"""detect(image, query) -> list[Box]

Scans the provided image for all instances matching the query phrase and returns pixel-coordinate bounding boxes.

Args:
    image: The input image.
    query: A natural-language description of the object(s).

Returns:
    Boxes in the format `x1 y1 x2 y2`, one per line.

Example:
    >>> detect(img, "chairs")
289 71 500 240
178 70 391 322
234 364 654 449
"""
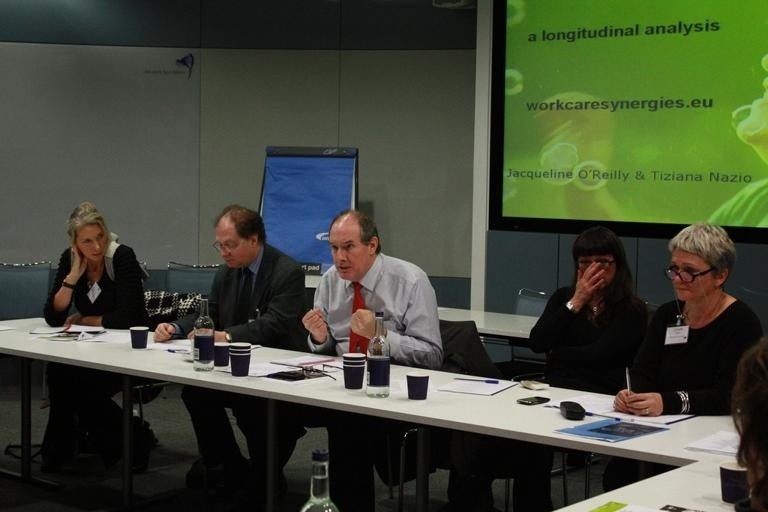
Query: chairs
0 261 768 512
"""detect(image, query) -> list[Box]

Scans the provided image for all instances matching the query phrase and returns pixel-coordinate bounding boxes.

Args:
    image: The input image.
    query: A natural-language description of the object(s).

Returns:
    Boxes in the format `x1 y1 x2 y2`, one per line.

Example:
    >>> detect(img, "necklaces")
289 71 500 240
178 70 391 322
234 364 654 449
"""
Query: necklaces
587 296 604 312
679 288 728 325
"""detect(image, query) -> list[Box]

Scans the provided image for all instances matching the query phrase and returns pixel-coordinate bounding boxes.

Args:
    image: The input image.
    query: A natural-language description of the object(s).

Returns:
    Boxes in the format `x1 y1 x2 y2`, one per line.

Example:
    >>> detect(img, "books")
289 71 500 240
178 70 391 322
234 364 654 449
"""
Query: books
34 318 107 340
273 348 362 372
437 366 520 396
147 329 263 355
545 377 693 444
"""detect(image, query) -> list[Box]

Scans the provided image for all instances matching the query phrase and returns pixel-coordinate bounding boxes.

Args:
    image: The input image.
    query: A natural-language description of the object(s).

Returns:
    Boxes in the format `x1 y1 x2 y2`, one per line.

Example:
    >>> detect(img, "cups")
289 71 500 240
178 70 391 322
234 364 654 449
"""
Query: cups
212 341 229 367
129 326 151 349
719 462 751 504
228 341 254 377
405 371 428 401
341 351 366 390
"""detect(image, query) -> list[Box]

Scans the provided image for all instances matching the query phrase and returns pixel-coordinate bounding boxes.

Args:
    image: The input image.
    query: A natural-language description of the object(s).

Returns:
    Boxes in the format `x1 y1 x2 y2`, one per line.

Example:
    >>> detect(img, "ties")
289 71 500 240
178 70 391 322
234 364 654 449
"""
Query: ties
349 282 369 354
233 267 253 330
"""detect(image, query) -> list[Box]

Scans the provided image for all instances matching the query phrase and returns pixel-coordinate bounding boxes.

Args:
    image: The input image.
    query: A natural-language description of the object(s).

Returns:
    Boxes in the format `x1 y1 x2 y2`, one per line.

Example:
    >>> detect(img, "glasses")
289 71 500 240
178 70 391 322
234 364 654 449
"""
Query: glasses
665 267 714 282
578 260 615 268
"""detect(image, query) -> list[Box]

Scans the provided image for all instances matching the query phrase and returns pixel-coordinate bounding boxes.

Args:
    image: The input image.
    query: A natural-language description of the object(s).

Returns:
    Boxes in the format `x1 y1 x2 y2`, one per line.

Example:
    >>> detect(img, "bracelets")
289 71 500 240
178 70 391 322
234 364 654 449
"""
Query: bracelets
60 281 77 290
677 388 690 414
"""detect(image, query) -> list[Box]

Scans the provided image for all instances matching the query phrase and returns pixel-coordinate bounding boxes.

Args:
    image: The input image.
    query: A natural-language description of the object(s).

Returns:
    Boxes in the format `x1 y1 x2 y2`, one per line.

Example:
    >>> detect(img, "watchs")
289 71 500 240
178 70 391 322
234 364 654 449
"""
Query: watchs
565 300 576 314
224 327 233 343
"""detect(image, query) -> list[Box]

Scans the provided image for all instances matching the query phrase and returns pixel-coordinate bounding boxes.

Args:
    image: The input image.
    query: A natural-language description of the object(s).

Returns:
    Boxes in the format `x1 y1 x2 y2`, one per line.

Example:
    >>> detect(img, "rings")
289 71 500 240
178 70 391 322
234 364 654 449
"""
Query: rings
355 314 361 322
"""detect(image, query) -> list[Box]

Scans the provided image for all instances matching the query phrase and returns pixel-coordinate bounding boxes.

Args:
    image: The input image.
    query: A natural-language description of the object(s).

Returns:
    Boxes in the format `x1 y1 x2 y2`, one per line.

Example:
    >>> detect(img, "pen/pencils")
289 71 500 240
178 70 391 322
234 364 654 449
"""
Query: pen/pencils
453 377 499 384
585 412 620 421
626 367 631 396
168 349 190 354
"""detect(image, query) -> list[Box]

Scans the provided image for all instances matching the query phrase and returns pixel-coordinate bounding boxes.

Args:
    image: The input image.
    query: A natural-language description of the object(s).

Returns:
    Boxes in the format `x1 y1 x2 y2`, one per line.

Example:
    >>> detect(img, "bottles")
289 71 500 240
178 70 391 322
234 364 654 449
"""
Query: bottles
192 295 214 373
299 449 338 512
365 311 391 399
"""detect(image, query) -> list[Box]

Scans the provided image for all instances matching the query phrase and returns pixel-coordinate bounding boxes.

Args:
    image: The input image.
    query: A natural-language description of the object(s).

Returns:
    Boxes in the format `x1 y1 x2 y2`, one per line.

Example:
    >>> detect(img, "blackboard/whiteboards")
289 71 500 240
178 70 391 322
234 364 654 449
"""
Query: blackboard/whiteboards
258 145 359 289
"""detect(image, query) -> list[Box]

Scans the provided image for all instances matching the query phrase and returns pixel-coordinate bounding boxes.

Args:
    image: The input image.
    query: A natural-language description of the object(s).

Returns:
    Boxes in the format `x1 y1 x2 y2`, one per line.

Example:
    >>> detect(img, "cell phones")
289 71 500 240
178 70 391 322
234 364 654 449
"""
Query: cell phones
517 396 550 405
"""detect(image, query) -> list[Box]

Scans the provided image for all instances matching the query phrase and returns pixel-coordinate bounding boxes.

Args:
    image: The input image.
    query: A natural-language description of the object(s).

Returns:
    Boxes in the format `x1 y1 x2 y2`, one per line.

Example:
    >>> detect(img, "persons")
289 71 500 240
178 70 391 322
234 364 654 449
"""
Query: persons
41 200 160 470
730 330 768 510
445 227 651 509
601 220 764 492
151 206 308 500
298 211 443 511
